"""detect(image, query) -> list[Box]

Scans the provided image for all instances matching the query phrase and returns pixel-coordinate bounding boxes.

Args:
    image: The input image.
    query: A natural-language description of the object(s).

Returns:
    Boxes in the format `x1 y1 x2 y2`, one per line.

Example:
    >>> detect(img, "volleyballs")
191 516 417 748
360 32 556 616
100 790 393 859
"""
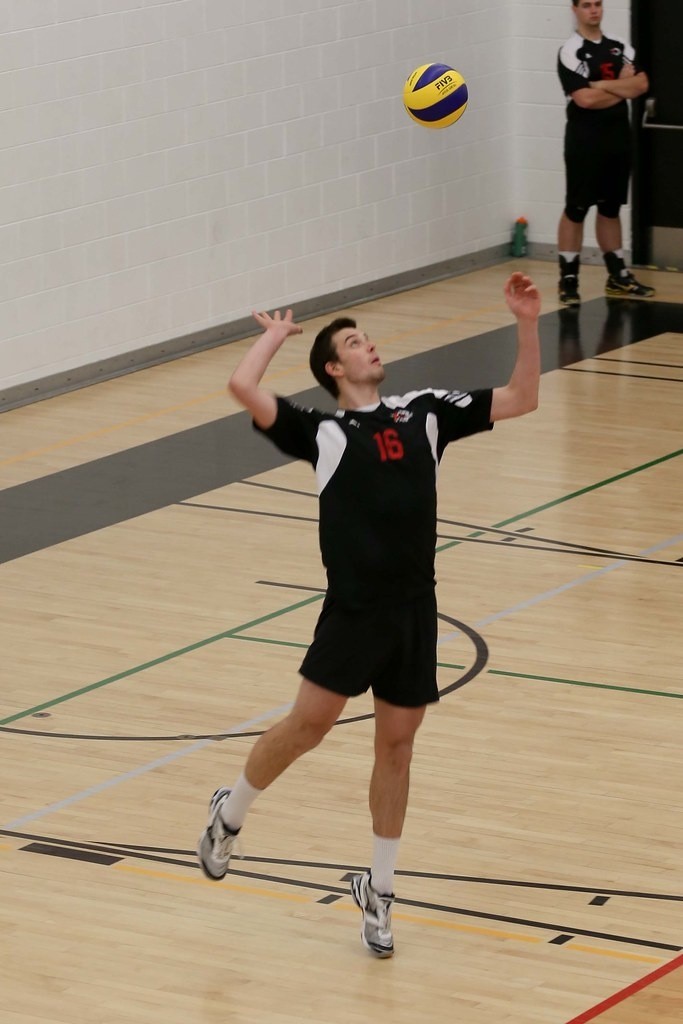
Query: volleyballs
401 61 470 131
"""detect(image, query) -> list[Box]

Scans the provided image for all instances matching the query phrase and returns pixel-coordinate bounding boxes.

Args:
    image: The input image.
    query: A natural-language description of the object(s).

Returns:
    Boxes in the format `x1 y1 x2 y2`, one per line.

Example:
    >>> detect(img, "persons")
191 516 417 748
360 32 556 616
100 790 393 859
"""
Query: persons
197 271 541 958
556 0 664 305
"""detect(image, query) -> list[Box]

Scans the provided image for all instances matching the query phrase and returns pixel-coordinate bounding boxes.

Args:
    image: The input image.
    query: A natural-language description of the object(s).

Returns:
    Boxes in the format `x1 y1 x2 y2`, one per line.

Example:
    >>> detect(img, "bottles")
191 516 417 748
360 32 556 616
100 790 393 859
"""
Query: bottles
512 218 528 256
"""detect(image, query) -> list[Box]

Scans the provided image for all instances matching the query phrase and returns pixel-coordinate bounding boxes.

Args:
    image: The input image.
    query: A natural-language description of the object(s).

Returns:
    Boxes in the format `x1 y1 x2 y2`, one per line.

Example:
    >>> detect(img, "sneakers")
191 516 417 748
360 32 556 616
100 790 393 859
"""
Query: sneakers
349 871 397 959
604 271 655 298
196 787 247 881
557 276 581 306
